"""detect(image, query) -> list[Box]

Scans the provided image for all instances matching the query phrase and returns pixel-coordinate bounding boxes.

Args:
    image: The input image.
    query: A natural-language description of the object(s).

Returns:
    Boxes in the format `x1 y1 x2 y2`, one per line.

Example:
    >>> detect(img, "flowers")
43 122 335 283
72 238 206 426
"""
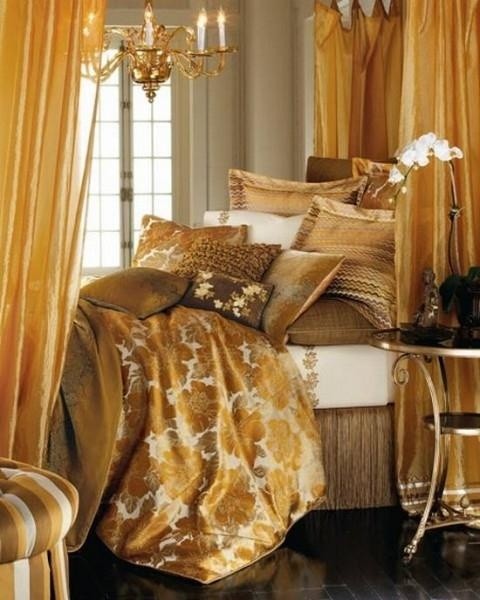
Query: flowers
386 138 464 274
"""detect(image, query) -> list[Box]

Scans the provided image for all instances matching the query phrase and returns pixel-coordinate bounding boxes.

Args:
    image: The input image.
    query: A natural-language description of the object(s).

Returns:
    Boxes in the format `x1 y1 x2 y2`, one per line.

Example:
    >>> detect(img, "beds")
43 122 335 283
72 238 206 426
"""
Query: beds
42 155 415 513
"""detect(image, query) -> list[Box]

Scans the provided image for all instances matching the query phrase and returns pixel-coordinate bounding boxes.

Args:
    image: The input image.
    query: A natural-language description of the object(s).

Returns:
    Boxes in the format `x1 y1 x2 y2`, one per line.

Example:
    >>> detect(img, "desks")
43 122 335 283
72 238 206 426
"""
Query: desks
368 326 480 559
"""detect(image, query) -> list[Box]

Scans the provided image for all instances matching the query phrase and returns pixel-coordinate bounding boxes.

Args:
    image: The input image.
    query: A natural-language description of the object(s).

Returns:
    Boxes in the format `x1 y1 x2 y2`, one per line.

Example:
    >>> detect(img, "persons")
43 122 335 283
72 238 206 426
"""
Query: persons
415 267 441 329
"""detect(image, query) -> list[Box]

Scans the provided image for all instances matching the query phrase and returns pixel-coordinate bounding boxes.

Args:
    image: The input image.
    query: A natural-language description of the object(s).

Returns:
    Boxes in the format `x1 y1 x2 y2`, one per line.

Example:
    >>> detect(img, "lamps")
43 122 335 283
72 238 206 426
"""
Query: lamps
80 0 237 101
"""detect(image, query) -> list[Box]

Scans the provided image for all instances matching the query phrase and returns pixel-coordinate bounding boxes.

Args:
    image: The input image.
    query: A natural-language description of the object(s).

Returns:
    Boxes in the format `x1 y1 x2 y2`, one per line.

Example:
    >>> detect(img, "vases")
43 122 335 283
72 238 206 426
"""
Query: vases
441 265 480 346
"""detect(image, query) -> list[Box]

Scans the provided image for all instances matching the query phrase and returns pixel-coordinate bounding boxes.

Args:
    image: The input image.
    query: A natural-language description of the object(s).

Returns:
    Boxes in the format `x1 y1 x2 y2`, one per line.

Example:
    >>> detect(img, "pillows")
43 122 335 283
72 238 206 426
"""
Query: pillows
170 235 285 280
180 272 278 328
200 157 397 247
248 248 349 344
79 265 189 324
290 195 399 334
130 214 248 278
